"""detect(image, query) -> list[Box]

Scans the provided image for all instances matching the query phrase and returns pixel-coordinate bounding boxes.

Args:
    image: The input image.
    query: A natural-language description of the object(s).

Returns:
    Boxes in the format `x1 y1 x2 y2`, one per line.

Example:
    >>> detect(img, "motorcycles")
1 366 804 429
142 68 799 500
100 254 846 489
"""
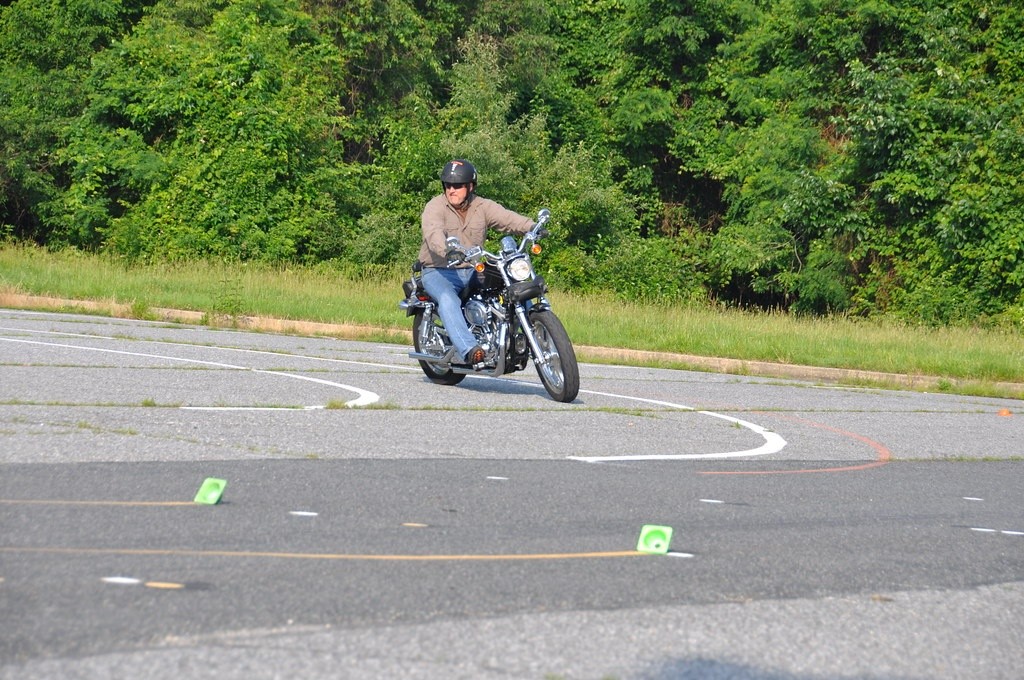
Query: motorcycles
401 207 580 404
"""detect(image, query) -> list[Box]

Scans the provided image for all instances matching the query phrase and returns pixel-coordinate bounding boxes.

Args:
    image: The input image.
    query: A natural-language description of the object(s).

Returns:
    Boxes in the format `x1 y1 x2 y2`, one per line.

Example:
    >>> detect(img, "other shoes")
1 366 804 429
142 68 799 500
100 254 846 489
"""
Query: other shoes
467 346 486 365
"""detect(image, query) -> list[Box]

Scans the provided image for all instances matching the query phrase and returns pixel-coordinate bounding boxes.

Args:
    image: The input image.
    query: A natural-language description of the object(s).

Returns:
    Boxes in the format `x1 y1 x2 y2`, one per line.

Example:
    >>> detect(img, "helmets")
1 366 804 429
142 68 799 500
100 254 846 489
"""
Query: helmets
441 159 477 190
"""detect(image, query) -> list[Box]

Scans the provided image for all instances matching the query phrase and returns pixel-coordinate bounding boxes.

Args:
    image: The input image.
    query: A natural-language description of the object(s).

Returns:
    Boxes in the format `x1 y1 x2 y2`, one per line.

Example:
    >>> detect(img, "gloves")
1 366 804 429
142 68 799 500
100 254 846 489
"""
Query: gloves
447 251 467 265
530 224 549 237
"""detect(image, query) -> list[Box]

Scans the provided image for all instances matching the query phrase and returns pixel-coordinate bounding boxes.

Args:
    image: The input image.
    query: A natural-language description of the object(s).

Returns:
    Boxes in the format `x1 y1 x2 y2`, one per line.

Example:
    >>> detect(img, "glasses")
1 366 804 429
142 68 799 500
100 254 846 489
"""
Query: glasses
443 182 466 189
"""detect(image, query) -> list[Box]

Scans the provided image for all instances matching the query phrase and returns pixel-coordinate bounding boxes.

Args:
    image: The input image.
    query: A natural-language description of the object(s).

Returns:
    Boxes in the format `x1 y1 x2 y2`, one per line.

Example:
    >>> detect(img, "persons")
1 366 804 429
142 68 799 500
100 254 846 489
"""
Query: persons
418 159 550 367
